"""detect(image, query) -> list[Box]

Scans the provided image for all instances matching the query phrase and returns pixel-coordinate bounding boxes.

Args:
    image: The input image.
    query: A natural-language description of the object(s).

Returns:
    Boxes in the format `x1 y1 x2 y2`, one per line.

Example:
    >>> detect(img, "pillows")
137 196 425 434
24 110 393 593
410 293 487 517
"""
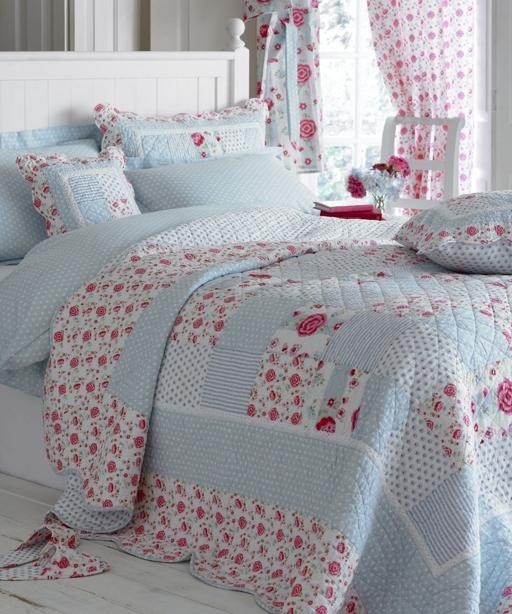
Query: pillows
389 189 511 273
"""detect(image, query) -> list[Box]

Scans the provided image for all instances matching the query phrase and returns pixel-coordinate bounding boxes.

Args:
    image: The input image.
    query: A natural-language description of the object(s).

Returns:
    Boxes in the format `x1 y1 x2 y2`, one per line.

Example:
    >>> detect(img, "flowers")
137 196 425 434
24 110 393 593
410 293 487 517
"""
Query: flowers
342 153 411 212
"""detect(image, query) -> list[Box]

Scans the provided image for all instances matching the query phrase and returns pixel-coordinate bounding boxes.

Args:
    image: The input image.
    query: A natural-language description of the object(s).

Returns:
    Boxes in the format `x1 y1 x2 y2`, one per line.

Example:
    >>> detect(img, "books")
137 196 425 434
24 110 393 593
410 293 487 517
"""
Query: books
311 199 374 213
320 208 382 220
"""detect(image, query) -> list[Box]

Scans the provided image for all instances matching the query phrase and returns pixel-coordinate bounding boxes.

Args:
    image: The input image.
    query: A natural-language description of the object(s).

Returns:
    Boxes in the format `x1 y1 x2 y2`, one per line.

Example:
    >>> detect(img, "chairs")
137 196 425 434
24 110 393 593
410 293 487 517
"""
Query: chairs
380 116 460 214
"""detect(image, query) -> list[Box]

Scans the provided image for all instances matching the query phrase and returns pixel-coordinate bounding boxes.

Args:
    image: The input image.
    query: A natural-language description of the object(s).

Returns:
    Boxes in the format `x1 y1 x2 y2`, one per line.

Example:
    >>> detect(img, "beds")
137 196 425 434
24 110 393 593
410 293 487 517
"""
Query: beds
1 16 512 612
